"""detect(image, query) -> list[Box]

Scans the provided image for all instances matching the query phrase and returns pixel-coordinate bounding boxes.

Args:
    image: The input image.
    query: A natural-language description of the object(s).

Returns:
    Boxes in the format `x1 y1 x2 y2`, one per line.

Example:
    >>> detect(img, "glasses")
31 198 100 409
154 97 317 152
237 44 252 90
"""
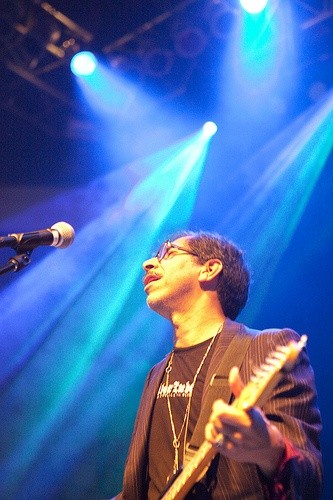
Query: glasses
155 240 208 261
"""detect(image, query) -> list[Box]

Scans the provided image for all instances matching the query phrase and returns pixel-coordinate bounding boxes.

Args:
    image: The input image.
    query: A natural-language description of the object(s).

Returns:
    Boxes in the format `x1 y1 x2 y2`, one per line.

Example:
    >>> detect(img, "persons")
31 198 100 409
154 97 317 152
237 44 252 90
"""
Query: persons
108 229 326 500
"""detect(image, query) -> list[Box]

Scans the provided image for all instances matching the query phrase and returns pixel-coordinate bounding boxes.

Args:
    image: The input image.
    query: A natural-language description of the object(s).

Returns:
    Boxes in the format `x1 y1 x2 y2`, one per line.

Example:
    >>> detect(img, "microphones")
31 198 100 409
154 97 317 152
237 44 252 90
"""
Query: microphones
0 222 75 249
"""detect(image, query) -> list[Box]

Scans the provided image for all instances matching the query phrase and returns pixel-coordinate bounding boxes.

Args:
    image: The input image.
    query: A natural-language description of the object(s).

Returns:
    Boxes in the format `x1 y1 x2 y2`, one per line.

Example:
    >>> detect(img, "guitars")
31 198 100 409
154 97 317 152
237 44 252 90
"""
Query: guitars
159 333 307 500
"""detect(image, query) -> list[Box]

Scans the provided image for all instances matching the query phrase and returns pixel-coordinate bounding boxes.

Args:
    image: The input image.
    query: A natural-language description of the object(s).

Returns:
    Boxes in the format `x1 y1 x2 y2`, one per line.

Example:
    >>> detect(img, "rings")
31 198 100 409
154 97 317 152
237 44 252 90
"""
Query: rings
215 434 224 447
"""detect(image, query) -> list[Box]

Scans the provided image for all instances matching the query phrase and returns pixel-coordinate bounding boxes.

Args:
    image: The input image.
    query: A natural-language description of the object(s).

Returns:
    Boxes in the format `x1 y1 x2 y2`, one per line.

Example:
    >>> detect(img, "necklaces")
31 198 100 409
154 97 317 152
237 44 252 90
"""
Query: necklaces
165 321 223 475
